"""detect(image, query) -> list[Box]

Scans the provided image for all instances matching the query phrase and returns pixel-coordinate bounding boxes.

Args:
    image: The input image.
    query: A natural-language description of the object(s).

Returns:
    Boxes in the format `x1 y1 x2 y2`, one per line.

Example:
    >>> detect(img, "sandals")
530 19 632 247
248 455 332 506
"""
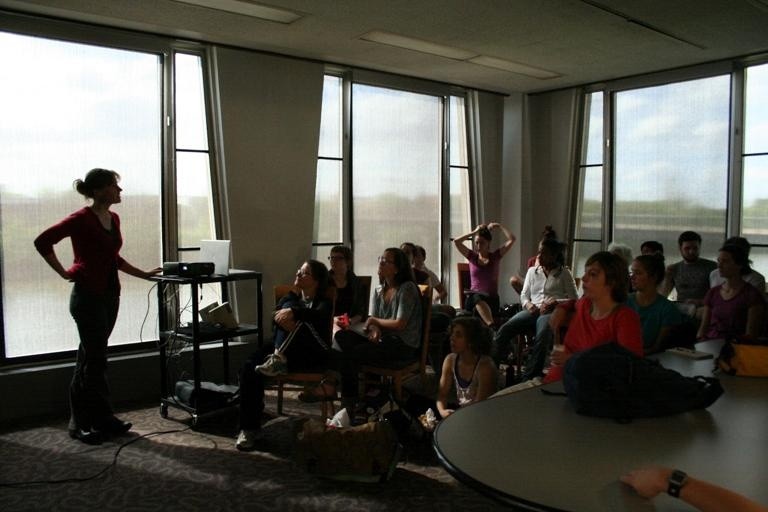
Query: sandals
297 375 337 404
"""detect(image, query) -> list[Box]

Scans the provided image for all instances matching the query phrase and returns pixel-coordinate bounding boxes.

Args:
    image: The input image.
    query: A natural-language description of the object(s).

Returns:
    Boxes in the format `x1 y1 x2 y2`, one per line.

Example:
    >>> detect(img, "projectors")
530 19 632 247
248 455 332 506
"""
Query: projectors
163 261 215 277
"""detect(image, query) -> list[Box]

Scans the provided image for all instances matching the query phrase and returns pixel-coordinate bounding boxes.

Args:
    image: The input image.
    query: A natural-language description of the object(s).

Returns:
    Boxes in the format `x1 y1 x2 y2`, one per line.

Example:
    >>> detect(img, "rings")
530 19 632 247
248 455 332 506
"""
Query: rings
283 315 289 320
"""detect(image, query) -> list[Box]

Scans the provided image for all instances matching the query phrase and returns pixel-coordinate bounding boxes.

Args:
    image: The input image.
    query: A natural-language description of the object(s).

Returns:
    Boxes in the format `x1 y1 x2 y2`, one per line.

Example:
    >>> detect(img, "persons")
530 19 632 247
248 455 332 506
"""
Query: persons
33 169 163 442
434 316 496 419
540 251 643 385
327 245 367 326
232 259 333 449
496 239 579 381
621 465 768 512
297 247 424 423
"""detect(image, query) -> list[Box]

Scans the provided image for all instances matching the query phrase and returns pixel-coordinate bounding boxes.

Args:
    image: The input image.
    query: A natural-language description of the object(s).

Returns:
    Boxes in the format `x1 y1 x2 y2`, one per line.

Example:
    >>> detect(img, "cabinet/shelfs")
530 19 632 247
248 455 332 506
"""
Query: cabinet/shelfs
149 267 267 436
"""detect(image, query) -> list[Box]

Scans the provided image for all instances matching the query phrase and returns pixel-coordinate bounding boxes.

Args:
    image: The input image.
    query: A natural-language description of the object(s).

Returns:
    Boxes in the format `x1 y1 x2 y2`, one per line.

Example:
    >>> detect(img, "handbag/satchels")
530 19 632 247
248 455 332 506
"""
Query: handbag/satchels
717 332 768 375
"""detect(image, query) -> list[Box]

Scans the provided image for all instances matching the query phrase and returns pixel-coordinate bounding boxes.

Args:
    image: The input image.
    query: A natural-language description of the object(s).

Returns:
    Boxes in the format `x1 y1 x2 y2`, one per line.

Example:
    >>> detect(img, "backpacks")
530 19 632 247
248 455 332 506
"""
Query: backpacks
564 343 724 422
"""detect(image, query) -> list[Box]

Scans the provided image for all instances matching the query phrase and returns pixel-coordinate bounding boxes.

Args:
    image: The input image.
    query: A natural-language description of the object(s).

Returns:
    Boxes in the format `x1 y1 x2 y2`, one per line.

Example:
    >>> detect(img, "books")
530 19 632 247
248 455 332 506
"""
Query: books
208 301 237 329
198 299 219 322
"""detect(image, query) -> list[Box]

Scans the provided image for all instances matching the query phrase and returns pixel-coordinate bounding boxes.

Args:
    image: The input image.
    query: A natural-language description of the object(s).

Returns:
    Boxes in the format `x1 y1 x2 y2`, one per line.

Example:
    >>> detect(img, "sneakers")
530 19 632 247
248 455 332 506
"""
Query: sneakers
238 429 267 452
254 356 289 378
68 421 133 444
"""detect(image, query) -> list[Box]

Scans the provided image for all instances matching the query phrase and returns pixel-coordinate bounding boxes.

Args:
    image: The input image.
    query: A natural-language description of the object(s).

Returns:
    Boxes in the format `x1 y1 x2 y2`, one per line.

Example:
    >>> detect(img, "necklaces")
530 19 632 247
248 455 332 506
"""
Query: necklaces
92 208 110 216
595 306 609 320
334 274 346 284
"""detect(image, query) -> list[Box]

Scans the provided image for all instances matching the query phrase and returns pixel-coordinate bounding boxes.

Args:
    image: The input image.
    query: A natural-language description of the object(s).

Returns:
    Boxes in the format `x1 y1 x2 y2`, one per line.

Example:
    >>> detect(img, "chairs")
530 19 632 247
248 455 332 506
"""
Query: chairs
666 300 710 345
256 262 535 430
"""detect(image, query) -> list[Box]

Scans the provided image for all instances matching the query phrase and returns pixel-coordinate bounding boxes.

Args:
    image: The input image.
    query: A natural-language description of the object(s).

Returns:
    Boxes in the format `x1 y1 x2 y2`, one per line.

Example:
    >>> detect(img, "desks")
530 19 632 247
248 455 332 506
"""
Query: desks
431 338 767 512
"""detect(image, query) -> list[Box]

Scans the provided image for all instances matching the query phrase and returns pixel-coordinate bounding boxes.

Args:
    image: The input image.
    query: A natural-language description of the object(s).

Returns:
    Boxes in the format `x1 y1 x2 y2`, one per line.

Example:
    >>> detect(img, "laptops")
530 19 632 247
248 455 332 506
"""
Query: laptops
199 240 255 276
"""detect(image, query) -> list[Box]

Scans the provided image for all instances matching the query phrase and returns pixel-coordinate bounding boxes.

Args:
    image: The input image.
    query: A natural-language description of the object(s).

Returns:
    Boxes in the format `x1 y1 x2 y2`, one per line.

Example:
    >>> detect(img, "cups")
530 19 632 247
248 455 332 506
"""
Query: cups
550 344 566 367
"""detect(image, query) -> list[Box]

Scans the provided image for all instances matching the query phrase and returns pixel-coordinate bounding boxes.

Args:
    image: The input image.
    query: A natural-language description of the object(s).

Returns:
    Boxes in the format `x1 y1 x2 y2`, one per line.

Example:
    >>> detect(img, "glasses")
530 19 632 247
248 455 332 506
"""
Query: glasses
296 269 313 276
327 255 345 261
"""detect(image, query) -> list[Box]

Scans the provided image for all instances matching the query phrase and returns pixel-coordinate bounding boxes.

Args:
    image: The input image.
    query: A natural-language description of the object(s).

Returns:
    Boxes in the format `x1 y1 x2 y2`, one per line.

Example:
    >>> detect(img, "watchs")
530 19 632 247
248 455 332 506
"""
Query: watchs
667 469 688 497
